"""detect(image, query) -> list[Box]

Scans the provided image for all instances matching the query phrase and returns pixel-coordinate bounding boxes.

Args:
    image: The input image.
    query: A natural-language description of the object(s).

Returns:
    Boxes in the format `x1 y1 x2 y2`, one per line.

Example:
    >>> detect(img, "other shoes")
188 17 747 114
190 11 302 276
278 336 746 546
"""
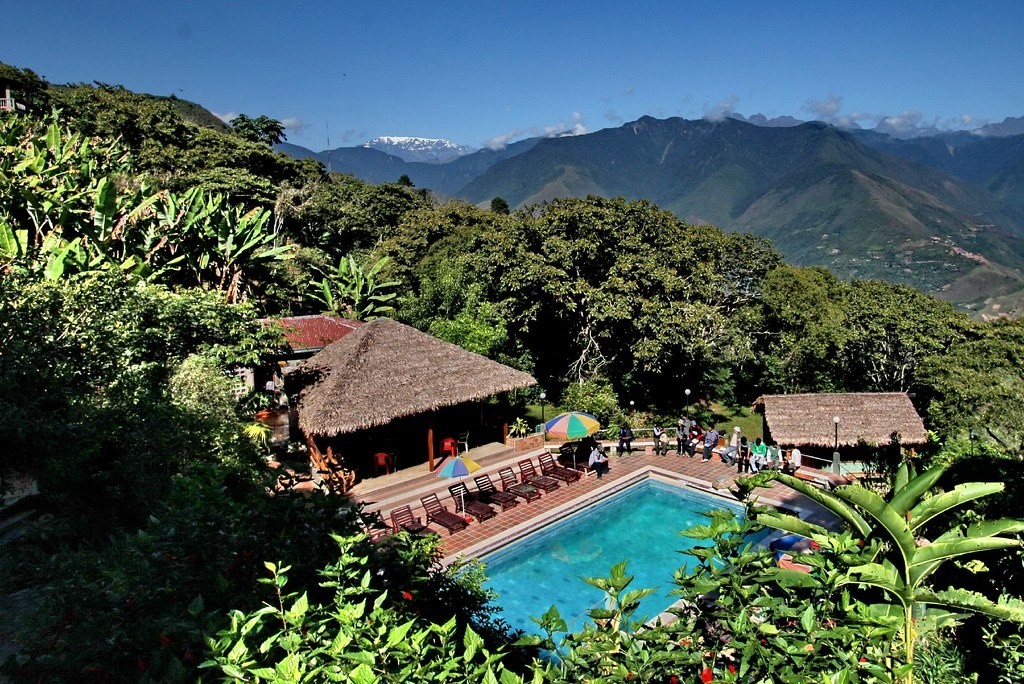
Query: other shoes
597 475 603 479
656 452 660 456
701 458 708 463
627 450 633 456
750 471 757 474
605 471 611 474
682 454 685 457
676 454 679 457
662 453 666 457
619 451 623 458
725 461 732 467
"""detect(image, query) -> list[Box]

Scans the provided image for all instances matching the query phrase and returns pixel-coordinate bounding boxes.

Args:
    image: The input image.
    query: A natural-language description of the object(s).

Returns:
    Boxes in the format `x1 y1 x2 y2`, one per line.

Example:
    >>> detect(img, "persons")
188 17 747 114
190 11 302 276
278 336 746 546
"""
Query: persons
653 419 802 474
588 444 607 479
618 420 632 456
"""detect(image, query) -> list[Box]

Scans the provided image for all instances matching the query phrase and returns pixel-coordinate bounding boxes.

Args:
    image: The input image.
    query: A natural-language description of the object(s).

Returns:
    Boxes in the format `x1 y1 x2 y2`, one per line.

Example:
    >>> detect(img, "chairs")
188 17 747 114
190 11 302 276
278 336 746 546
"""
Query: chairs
538 451 580 486
448 482 495 523
559 445 596 476
474 474 517 512
365 514 396 545
373 452 392 475
518 458 559 494
314 455 354 490
391 504 437 536
457 431 469 453
498 467 540 504
438 437 456 457
420 493 466 535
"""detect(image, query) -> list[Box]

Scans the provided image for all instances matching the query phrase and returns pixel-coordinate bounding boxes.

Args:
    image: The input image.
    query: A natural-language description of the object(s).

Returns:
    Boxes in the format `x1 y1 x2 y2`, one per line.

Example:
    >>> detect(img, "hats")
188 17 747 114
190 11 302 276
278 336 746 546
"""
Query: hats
690 420 697 426
677 419 684 425
733 425 741 433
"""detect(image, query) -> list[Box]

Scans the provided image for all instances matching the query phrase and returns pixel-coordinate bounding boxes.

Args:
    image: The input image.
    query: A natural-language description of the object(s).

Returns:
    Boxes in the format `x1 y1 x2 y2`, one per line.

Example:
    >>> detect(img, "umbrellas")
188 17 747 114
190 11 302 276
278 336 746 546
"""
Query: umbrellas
436 457 481 522
545 410 601 473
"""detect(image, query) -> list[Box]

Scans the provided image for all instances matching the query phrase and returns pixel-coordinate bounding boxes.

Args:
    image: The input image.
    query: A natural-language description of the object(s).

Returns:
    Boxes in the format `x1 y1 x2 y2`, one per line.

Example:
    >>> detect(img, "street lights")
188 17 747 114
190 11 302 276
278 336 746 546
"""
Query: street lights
539 392 547 423
628 400 635 430
832 416 840 452
683 389 691 419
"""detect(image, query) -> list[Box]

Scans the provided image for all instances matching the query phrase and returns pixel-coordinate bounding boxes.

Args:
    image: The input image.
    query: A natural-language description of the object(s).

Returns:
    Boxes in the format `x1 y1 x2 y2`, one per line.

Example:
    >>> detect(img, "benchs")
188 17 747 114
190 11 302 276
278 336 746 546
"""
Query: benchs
543 438 849 489
